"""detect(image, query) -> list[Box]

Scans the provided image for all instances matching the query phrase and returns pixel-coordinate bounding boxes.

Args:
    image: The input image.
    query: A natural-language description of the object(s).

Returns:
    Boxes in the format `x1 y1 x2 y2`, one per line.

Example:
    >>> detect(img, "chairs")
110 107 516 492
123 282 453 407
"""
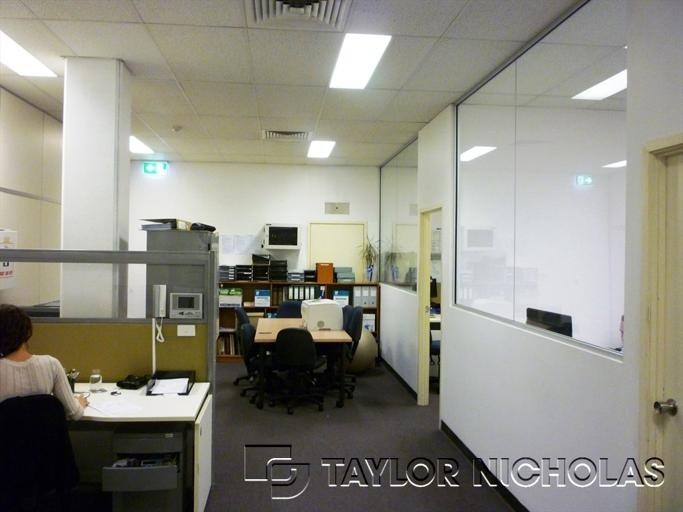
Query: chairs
0 393 79 511
232 299 363 415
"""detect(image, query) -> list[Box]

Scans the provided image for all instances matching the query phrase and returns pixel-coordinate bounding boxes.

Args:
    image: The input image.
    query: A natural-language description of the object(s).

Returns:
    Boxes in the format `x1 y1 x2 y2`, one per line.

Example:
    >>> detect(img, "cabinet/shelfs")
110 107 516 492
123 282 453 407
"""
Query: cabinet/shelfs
100 429 184 511
216 281 380 364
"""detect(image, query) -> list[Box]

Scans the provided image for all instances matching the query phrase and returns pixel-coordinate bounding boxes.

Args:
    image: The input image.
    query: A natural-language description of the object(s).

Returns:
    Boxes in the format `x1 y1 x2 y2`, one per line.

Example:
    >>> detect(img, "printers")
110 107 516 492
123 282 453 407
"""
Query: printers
301 298 343 330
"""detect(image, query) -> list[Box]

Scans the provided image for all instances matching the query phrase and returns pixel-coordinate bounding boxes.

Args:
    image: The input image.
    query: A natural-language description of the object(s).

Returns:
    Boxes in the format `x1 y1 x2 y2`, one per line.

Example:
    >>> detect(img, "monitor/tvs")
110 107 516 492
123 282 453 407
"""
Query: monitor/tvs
264 224 302 250
527 307 572 336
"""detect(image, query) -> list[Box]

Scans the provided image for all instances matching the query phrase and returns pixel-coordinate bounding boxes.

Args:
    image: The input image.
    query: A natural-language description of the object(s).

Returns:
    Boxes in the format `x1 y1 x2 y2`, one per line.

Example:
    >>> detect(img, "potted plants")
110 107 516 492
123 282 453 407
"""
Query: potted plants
354 232 379 282
383 237 400 283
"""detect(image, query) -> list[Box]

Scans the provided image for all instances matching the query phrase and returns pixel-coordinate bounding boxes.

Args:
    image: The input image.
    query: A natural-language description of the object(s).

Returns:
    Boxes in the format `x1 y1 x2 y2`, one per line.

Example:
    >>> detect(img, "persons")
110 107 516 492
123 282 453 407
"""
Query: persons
0 304 90 423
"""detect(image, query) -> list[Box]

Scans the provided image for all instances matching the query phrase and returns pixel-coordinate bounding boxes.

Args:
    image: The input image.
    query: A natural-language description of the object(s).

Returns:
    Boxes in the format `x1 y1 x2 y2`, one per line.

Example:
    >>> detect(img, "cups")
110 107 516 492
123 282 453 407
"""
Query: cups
67 377 75 394
88 374 103 391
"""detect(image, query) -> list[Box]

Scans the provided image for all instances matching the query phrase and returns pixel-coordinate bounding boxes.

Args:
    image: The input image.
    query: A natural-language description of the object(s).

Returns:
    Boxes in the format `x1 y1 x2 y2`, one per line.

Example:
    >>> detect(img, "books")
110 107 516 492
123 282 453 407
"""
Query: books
217 333 236 356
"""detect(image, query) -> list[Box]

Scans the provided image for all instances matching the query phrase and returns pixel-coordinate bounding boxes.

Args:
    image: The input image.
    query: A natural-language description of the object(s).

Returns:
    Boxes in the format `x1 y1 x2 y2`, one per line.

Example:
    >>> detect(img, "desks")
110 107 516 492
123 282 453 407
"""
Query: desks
51 379 212 511
